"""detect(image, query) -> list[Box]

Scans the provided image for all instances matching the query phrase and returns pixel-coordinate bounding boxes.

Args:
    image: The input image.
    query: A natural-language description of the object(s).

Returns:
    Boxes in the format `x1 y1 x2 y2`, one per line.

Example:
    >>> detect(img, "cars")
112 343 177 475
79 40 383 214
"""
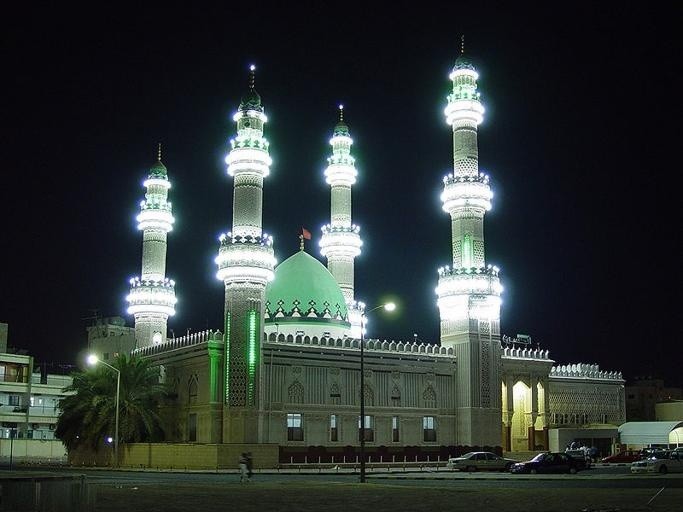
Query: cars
507 450 577 476
560 445 683 476
447 450 521 473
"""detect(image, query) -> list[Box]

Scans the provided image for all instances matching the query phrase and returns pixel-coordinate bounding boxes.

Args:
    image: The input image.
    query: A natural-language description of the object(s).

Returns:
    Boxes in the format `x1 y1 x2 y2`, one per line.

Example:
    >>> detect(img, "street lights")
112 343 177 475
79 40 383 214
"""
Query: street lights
5 422 14 471
356 302 398 484
86 353 122 470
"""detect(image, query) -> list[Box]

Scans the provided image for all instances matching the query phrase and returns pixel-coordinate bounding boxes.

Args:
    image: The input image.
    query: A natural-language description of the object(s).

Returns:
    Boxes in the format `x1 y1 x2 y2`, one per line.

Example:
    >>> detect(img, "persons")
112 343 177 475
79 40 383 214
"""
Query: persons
238 451 248 483
564 440 597 465
246 451 253 482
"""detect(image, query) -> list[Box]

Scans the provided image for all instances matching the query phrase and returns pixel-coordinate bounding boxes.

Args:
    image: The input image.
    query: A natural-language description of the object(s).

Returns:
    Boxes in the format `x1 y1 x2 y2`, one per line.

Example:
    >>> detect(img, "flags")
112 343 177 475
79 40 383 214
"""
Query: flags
303 228 312 240
